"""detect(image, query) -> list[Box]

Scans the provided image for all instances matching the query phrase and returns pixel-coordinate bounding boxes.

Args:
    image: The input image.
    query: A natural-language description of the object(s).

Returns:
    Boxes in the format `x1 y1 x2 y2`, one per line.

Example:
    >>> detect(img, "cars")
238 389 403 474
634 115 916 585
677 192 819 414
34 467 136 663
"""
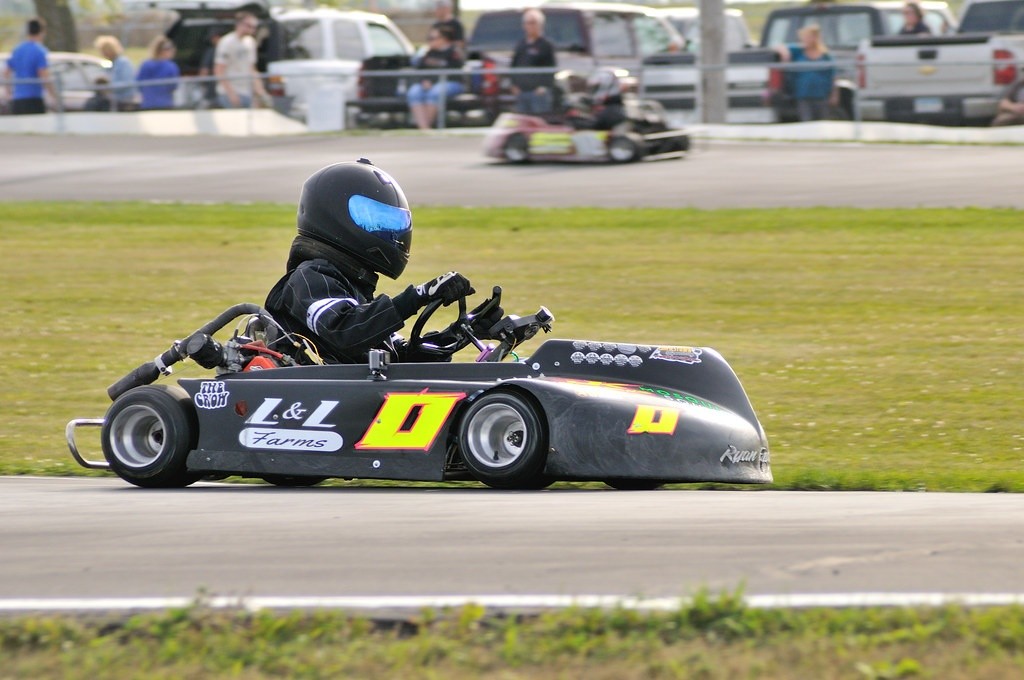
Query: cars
0 52 142 110
658 9 750 54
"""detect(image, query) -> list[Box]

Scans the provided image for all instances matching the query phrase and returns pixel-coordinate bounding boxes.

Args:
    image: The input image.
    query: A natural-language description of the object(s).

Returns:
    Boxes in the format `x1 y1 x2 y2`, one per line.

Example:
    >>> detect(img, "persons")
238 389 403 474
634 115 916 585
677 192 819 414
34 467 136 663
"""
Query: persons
537 67 634 129
900 3 930 34
265 157 505 365
994 66 1024 126
84 77 111 112
137 39 179 110
198 29 226 77
769 23 838 122
98 36 135 112
5 20 60 115
214 12 272 109
508 9 555 114
407 0 466 128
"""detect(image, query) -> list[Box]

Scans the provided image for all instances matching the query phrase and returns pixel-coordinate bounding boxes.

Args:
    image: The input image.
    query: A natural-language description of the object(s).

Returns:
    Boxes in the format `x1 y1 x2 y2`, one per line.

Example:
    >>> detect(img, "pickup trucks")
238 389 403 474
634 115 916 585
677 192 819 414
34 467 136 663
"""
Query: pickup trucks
854 0 1024 121
639 0 957 107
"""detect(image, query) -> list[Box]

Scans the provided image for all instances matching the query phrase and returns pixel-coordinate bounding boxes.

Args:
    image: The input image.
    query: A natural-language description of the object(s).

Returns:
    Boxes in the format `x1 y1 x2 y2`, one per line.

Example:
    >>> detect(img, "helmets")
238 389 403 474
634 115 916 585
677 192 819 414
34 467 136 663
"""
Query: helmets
584 65 628 102
297 158 412 281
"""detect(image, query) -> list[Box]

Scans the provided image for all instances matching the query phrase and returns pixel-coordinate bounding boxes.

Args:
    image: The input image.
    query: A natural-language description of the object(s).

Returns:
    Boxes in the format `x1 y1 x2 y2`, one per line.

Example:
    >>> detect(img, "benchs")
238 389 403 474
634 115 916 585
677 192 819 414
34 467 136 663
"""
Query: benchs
342 50 560 129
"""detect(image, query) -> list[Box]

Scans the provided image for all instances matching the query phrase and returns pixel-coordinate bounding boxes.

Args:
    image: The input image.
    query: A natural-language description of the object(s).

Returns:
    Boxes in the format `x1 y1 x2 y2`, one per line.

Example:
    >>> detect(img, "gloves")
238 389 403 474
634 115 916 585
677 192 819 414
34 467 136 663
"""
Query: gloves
460 298 503 340
416 272 470 306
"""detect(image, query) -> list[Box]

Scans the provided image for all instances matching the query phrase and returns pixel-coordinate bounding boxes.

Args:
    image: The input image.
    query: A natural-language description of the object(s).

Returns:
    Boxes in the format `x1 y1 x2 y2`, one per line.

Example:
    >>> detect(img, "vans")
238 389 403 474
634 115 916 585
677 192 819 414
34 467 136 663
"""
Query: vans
145 0 415 132
471 4 690 101
